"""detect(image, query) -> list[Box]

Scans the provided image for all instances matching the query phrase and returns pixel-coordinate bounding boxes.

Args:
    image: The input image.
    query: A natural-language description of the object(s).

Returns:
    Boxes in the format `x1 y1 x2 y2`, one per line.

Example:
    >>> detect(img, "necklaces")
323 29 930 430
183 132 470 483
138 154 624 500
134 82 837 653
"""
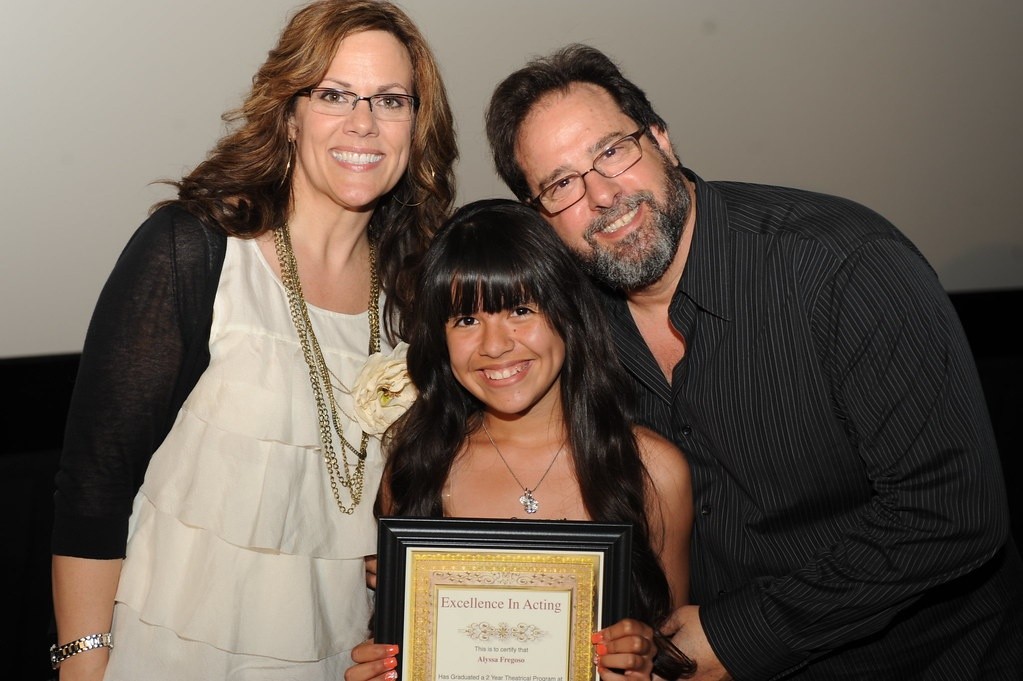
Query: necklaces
478 422 567 514
270 216 382 516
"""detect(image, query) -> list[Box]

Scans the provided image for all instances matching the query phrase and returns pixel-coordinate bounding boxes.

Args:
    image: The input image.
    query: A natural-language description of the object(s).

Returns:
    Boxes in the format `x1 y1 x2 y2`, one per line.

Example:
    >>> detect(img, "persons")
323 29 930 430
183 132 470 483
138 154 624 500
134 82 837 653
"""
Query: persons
343 200 692 681
487 43 1023 681
51 0 460 681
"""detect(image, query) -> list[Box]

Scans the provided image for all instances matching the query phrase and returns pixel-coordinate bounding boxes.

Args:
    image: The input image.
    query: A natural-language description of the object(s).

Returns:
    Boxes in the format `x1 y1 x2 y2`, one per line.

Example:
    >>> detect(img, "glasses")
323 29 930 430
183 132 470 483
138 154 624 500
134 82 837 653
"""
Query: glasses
530 124 648 217
300 86 420 122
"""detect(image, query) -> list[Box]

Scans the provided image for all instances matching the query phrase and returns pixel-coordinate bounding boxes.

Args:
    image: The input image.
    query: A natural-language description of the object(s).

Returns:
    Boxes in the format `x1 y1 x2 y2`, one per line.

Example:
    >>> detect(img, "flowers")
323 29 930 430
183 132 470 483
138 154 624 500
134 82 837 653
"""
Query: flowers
350 341 420 445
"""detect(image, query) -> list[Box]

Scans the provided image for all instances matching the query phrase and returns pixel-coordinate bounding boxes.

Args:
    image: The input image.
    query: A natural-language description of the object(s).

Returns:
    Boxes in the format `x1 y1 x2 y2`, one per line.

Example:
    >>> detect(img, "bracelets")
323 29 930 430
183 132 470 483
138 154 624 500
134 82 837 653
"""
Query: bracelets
50 633 114 670
